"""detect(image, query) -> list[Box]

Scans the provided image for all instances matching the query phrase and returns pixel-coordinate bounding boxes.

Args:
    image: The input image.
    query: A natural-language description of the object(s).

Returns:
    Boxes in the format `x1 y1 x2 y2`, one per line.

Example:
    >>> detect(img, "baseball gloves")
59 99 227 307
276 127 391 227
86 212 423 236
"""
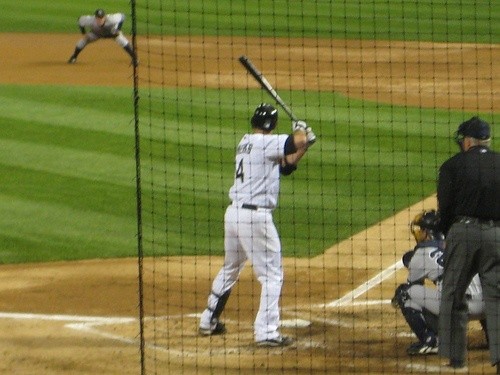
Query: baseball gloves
391 283 411 309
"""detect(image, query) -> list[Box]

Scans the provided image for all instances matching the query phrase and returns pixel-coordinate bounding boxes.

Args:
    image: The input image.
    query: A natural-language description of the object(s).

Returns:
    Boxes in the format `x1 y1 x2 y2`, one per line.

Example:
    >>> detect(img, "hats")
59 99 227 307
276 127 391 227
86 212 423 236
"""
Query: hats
95 9 105 17
457 116 492 140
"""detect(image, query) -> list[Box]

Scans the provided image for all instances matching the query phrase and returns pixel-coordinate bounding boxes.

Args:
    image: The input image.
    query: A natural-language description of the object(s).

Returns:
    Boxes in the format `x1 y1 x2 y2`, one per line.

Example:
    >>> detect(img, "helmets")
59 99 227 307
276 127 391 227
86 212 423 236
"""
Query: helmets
410 207 447 244
250 102 279 130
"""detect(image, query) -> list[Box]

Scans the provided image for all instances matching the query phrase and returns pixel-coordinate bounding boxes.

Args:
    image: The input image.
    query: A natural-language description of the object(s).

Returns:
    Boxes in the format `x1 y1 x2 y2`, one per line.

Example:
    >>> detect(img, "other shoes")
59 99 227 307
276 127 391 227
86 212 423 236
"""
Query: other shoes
403 341 441 357
67 55 76 64
198 321 225 334
256 332 293 347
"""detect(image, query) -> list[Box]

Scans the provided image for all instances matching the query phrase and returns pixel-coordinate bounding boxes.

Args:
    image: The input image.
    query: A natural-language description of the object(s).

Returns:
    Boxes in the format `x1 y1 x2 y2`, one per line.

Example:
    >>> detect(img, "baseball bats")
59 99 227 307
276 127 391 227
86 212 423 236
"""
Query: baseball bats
237 54 316 144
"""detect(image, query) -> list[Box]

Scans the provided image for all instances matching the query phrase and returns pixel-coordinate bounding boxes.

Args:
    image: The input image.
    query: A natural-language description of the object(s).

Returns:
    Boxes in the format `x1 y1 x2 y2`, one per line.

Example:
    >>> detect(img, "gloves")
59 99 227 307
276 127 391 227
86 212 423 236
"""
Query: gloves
304 126 317 145
292 119 307 130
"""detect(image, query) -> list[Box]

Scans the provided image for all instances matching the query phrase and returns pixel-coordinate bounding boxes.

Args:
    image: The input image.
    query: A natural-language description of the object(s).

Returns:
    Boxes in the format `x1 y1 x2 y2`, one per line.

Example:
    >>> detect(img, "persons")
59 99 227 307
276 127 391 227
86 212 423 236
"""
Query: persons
67 9 140 67
197 101 316 348
392 209 491 357
434 116 500 375
